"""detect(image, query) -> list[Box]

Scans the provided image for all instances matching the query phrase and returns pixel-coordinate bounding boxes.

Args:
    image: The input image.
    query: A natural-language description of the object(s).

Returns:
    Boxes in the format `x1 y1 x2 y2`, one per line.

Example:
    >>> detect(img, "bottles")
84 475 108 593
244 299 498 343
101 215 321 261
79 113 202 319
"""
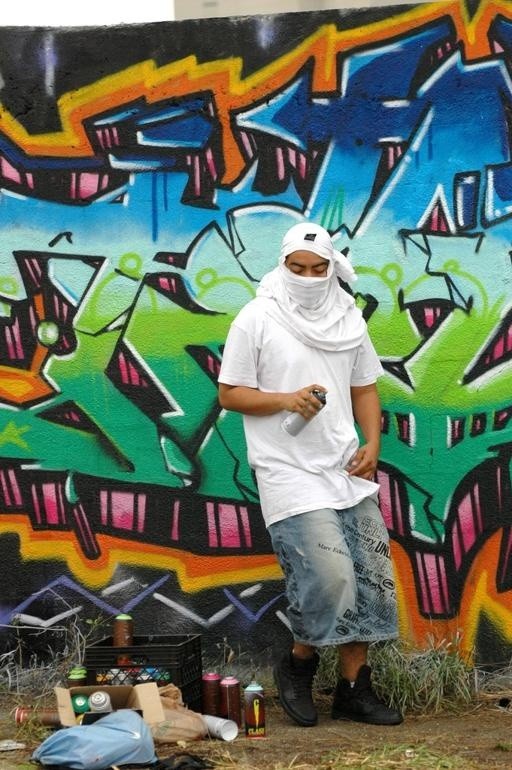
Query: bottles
112 612 134 651
198 668 266 743
15 708 61 726
281 389 328 437
70 659 170 713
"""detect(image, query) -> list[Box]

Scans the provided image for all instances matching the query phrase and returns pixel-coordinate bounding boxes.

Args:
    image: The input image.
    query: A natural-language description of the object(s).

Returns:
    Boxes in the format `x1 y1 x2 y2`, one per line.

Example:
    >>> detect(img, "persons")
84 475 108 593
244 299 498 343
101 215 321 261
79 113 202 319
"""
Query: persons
214 219 408 728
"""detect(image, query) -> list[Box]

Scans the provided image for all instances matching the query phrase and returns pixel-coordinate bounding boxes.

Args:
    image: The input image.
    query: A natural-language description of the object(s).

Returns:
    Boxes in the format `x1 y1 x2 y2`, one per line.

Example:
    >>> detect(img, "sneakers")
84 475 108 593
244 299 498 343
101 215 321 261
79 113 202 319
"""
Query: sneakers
329 662 405 727
271 643 322 728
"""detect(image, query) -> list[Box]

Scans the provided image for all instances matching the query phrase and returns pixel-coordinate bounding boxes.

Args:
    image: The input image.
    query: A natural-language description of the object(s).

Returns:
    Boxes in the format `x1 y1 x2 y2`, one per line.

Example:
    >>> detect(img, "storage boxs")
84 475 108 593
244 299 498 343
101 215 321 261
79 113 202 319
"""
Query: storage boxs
54 679 165 729
